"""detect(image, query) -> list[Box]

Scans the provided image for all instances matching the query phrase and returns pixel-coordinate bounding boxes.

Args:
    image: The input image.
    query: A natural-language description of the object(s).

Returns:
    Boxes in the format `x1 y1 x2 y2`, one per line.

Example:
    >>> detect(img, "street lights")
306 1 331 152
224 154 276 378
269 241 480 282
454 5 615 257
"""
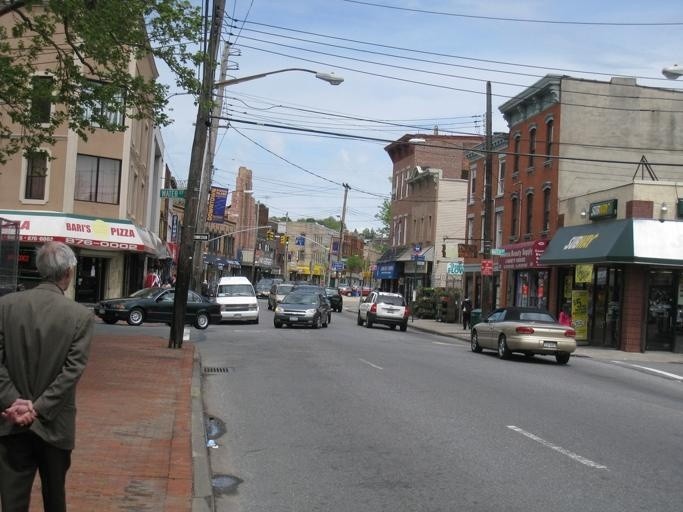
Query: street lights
662 63 683 80
192 68 345 294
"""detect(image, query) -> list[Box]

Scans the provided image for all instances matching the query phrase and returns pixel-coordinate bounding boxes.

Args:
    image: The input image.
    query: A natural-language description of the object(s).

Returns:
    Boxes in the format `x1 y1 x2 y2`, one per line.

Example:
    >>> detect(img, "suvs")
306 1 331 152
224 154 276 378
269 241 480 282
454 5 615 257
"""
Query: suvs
358 289 410 331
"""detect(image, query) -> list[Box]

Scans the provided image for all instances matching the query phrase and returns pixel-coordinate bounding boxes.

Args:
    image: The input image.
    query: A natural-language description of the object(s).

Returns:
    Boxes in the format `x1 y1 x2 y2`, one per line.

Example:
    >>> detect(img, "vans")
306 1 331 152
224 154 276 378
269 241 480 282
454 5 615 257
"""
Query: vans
215 276 260 324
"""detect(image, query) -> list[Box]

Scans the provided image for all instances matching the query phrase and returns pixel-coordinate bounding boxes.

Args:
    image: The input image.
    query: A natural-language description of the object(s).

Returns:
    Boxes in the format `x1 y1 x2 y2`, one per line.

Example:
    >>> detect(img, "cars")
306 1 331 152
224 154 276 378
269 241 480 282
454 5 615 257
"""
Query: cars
339 284 374 296
256 284 342 329
94 287 222 329
470 307 578 365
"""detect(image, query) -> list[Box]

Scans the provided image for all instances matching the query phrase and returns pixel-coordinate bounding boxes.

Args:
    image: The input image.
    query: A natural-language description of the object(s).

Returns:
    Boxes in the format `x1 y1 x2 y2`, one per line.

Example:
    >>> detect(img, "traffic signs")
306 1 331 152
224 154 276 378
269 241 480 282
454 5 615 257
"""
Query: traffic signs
160 189 186 199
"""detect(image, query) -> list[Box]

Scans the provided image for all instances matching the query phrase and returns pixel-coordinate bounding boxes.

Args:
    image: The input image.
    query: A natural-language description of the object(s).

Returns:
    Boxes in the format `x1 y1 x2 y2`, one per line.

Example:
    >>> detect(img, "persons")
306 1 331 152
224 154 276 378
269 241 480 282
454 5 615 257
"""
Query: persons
558 304 570 326
461 296 471 330
0 241 94 511
